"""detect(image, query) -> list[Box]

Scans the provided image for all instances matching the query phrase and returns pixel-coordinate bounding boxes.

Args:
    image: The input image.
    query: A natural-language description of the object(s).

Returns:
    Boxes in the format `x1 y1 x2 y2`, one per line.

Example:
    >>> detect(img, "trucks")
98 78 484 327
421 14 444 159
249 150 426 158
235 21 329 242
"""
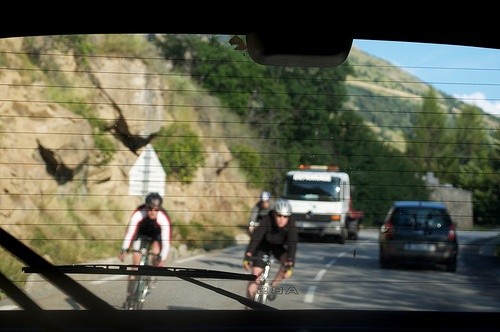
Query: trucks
277 164 365 244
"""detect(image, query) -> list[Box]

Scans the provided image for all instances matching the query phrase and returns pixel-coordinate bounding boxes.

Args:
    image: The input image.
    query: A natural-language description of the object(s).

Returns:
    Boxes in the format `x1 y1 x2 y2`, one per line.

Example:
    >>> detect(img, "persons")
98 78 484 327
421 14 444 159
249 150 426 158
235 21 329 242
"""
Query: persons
121 192 171 309
243 199 297 310
247 191 273 237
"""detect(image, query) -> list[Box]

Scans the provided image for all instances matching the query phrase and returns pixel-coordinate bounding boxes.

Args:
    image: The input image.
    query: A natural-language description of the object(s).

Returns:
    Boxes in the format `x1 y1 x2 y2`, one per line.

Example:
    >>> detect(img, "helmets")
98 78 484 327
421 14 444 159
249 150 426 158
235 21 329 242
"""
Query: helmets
260 191 270 201
146 192 162 208
274 198 292 216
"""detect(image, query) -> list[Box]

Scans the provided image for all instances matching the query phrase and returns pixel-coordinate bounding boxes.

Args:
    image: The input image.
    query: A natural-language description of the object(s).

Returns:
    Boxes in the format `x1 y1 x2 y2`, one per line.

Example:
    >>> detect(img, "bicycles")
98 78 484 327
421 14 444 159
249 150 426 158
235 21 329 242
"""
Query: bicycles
242 254 287 307
119 247 159 310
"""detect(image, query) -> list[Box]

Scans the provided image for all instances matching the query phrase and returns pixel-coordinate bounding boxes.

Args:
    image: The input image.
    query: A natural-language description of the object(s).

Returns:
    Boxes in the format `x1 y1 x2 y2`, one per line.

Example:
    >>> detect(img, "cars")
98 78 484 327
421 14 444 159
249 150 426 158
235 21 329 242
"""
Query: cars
377 201 459 272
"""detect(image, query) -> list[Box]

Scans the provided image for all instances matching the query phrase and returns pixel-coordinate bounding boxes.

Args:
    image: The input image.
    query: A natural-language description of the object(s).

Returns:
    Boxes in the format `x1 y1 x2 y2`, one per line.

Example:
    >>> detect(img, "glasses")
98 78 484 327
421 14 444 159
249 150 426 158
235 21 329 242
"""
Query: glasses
277 214 288 218
147 209 159 211
